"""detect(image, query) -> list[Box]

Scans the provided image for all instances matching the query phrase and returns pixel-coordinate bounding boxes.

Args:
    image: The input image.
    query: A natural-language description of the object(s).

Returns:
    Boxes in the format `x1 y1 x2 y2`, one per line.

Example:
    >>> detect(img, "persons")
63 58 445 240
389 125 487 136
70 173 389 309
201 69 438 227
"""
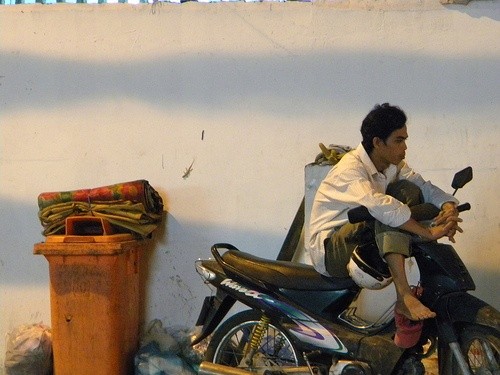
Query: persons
306 102 463 322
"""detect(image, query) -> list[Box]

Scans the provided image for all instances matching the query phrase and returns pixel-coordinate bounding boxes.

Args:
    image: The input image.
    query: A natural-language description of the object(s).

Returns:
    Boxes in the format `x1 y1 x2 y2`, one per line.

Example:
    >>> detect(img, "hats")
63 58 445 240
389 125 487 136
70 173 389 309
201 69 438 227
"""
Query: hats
394 287 426 349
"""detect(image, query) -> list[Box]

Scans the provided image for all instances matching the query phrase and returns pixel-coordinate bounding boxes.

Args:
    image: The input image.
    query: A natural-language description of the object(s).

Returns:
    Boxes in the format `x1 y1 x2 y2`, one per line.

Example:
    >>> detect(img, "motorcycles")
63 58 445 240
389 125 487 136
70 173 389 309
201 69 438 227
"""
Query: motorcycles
188 166 500 375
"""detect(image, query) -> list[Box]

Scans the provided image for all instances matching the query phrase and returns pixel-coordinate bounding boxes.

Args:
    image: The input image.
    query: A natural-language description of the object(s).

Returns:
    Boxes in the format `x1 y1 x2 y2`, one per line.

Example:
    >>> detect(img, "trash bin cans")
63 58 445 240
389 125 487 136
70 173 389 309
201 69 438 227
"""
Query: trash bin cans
30 215 150 375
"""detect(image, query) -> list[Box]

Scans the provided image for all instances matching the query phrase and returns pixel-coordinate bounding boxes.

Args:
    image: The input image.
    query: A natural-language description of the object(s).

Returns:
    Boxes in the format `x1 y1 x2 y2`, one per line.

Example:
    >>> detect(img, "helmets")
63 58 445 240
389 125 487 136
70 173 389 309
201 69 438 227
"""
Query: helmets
348 246 394 290
